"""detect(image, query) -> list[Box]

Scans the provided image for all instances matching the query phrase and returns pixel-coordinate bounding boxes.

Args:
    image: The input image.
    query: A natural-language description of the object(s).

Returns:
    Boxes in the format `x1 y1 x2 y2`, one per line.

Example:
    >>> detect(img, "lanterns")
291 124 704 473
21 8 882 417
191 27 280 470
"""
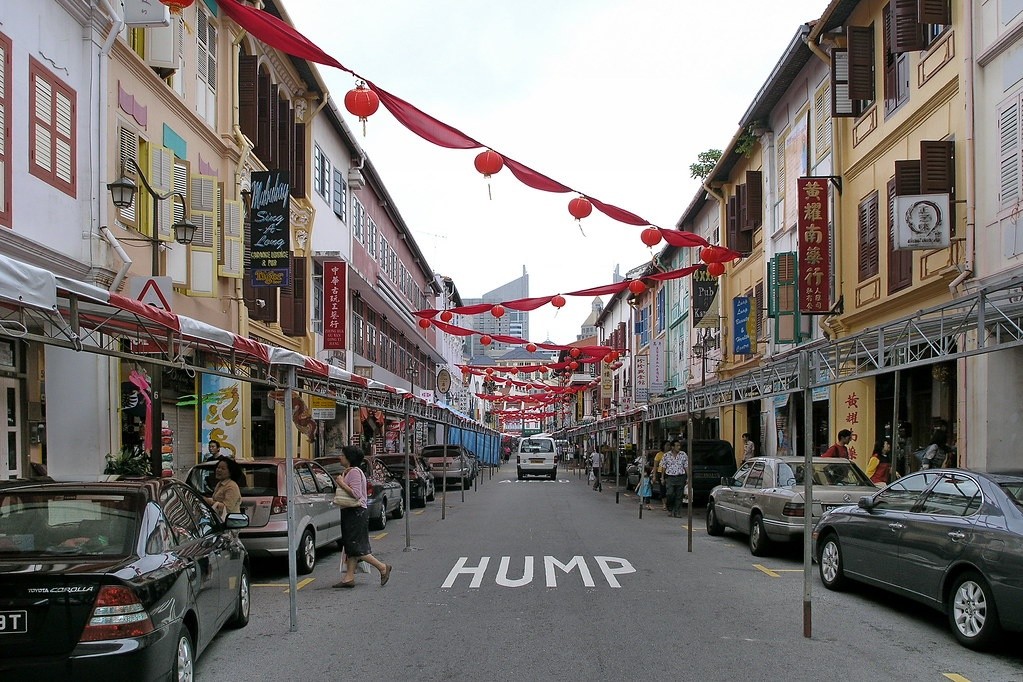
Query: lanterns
568 196 592 238
641 228 662 256
160 0 196 34
419 247 726 423
344 86 379 137
474 150 504 200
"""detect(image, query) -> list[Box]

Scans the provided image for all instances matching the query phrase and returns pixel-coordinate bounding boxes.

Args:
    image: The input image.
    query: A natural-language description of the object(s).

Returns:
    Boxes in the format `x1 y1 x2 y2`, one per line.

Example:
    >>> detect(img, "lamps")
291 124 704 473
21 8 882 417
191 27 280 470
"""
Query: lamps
667 387 677 395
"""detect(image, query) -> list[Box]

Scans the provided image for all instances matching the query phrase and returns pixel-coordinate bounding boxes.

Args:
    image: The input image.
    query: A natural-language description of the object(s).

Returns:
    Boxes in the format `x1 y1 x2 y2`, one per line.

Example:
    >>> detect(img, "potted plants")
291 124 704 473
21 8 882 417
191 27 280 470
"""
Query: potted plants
100 443 153 485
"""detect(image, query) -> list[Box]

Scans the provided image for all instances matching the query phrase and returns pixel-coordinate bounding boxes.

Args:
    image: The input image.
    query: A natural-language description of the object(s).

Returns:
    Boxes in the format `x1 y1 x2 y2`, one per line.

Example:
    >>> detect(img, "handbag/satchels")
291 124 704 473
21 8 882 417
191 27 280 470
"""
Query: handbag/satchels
340 547 371 574
204 564 213 582
334 468 363 508
913 445 938 467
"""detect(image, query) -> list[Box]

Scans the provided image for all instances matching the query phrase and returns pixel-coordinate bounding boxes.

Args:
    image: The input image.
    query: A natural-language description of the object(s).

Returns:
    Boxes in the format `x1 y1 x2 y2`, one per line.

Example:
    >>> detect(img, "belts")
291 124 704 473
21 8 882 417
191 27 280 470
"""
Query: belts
668 475 685 477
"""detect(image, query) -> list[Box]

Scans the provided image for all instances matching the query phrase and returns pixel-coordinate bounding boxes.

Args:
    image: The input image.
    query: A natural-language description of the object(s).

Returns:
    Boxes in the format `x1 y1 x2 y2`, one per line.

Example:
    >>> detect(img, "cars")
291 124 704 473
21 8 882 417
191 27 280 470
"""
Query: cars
625 449 661 495
184 457 343 575
313 457 406 529
705 455 882 558
811 466 1023 651
0 478 252 682
375 454 436 507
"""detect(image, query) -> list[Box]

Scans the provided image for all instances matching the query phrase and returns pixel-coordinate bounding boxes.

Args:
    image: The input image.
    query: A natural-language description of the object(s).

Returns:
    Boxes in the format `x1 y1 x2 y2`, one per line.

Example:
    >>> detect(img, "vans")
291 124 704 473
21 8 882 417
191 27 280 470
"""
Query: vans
516 436 558 480
680 440 737 504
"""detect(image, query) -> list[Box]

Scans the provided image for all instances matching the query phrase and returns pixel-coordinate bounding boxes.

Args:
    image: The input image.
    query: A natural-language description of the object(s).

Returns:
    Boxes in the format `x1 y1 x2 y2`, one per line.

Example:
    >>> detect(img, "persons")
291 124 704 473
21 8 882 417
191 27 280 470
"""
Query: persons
919 430 957 472
822 429 851 484
330 446 393 589
555 433 755 518
200 440 243 540
864 440 902 489
502 441 519 464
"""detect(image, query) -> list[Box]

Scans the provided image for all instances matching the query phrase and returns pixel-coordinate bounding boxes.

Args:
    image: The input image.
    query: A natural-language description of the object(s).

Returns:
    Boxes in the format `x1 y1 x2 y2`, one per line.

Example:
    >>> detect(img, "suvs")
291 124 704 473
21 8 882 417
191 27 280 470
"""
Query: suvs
421 444 475 490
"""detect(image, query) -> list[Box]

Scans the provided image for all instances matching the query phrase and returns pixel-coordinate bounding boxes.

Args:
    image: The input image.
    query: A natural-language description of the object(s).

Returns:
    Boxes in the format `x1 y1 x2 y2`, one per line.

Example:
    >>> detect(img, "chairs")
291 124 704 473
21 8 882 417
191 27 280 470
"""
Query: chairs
253 473 277 494
0 510 131 556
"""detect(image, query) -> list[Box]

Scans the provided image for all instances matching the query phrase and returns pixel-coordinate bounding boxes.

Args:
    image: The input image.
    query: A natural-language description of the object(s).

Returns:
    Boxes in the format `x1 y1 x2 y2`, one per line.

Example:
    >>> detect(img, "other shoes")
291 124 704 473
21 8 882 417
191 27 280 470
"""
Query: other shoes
332 580 356 588
642 504 654 511
662 504 667 511
381 565 392 586
592 488 602 491
668 512 682 518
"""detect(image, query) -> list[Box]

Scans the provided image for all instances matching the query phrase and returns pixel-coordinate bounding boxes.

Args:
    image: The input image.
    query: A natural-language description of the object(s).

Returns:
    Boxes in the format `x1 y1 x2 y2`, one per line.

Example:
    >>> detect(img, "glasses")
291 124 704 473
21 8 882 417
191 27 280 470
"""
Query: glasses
337 454 345 458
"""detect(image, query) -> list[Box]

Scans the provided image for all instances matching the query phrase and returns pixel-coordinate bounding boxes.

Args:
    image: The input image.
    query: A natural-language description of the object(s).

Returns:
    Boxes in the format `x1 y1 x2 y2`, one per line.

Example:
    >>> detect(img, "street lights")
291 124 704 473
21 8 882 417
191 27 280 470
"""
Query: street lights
622 379 633 449
691 325 715 440
107 154 198 476
406 363 419 454
